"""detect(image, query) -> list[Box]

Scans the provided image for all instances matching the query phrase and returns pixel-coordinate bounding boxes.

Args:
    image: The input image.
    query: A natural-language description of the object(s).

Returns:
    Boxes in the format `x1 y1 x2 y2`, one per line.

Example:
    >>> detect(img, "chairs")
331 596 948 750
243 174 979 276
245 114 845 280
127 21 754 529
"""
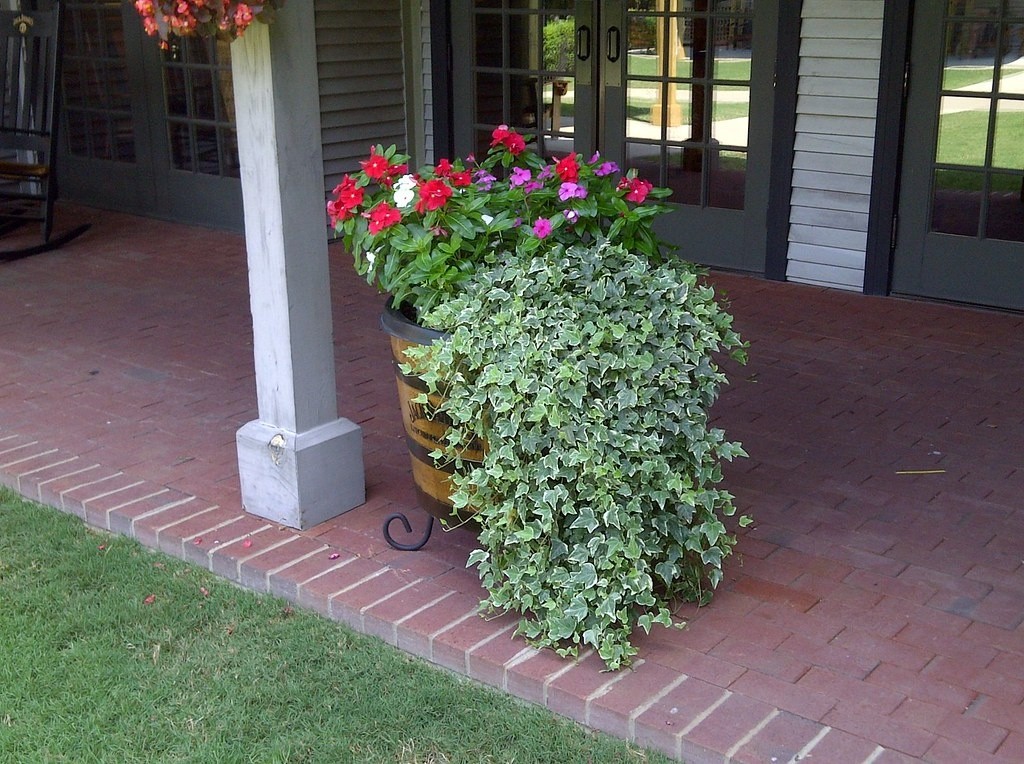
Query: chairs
0 3 92 262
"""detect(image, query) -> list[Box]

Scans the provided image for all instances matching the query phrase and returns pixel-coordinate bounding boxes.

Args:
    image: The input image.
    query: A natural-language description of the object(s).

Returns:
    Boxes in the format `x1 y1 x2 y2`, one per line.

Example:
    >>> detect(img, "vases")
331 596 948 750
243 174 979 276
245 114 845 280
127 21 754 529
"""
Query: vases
378 295 500 531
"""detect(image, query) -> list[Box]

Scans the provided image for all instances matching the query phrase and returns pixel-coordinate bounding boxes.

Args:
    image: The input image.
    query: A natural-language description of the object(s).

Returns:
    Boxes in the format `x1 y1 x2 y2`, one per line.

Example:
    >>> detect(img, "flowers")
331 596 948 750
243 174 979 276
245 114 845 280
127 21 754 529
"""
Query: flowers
134 0 285 50
325 125 676 322
